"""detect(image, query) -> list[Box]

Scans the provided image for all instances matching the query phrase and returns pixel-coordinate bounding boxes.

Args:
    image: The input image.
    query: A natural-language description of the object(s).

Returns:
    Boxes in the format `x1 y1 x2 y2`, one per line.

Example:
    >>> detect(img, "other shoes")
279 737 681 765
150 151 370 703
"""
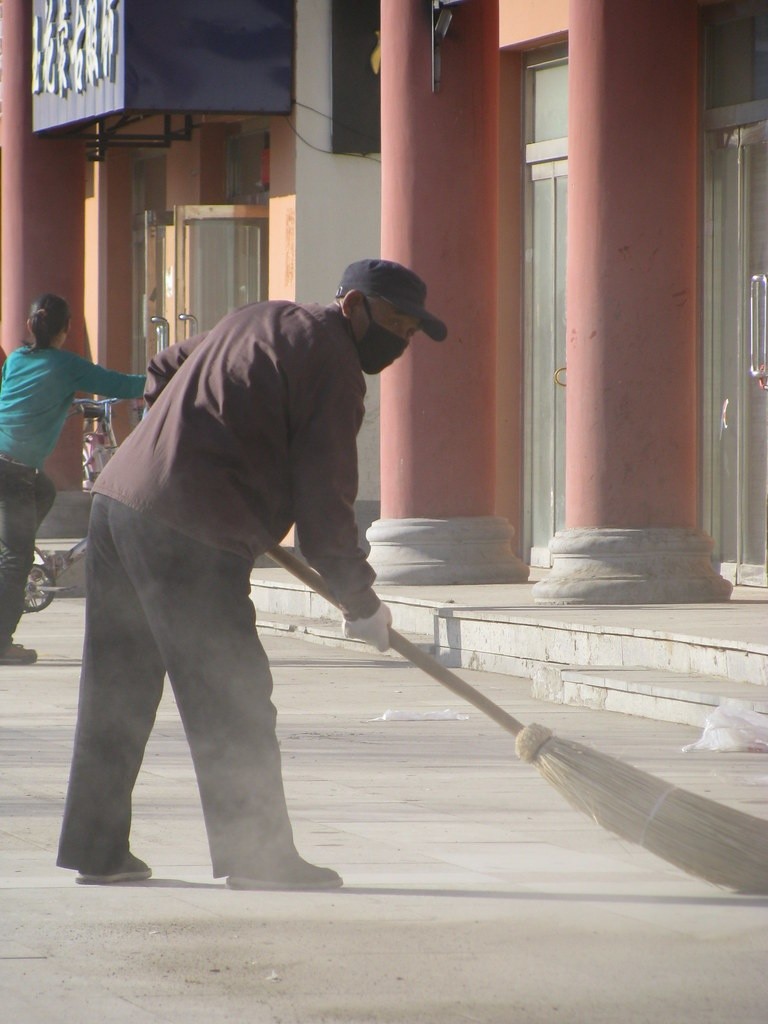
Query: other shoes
0 643 37 664
75 849 152 884
225 846 343 892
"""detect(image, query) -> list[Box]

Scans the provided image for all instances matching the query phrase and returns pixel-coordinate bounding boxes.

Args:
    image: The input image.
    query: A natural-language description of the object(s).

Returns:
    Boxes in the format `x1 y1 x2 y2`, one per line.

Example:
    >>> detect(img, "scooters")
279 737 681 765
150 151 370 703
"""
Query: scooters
14 396 139 615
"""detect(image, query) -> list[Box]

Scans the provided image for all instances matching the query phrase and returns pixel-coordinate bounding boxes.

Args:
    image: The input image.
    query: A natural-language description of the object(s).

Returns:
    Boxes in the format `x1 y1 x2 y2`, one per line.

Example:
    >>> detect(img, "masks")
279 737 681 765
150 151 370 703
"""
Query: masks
347 294 410 375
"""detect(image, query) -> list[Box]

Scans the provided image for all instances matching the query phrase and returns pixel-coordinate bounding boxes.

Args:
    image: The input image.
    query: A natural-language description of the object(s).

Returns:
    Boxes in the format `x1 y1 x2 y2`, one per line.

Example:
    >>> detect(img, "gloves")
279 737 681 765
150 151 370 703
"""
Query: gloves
342 599 392 650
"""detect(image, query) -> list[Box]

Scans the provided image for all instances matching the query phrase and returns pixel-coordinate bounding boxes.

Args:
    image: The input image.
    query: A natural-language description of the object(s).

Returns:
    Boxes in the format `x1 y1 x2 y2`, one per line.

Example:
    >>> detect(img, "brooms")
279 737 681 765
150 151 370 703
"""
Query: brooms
266 531 768 897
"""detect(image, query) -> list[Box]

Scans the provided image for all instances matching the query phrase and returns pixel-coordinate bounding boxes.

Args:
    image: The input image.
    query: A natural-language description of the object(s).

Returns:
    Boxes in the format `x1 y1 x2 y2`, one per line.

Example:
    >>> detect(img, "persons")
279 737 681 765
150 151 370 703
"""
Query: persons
0 293 149 665
57 258 447 890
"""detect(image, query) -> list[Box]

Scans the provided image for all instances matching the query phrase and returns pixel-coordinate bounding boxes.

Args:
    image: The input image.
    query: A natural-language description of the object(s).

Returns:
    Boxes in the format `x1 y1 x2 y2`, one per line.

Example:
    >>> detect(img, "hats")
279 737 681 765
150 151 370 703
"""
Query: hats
336 259 447 342
